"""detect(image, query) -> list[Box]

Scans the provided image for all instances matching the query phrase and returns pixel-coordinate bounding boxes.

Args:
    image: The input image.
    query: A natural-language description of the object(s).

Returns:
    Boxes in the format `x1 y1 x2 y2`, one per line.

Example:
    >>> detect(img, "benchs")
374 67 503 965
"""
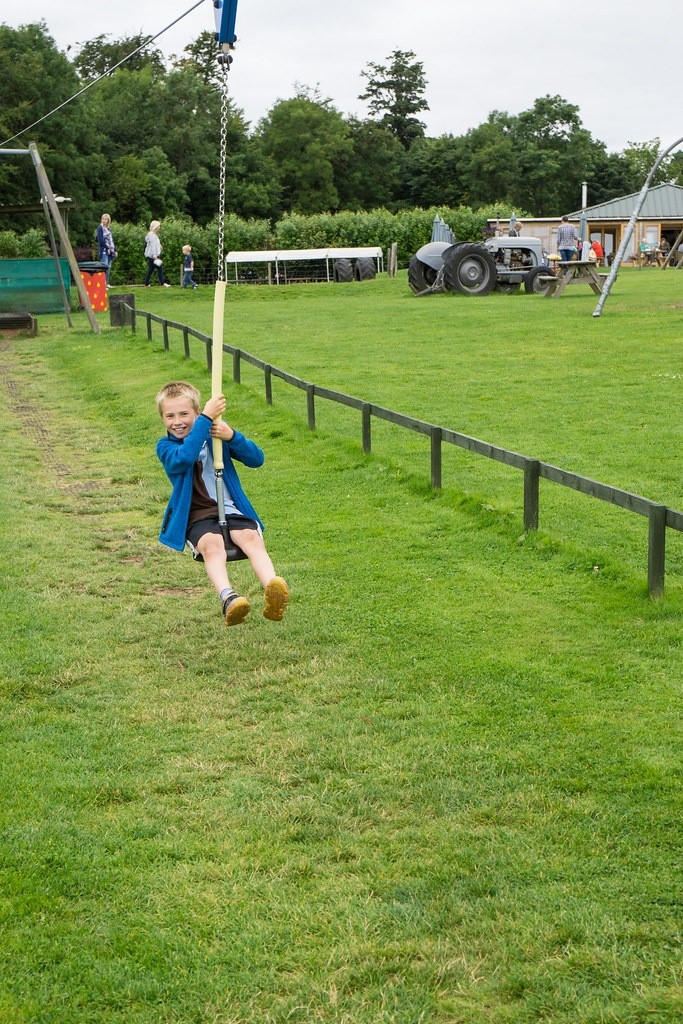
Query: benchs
581 273 619 283
631 256 674 261
537 275 558 285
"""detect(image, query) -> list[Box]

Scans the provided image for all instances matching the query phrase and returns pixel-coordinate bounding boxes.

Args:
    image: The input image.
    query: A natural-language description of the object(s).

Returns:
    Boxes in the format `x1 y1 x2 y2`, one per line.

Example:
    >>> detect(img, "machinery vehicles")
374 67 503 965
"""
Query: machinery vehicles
407 236 558 299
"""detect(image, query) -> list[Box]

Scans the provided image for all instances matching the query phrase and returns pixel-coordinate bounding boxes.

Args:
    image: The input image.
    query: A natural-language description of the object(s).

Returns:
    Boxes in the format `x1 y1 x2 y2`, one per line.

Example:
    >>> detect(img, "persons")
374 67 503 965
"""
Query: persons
637 238 655 268
578 240 606 268
144 221 171 287
557 217 581 276
509 222 523 236
657 238 673 267
97 213 118 290
157 381 289 626
181 245 198 289
678 244 683 267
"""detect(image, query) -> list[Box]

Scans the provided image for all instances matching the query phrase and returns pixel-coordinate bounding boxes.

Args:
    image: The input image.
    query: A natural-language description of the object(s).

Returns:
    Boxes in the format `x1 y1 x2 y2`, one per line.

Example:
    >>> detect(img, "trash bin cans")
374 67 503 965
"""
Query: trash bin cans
606 254 616 266
77 261 109 311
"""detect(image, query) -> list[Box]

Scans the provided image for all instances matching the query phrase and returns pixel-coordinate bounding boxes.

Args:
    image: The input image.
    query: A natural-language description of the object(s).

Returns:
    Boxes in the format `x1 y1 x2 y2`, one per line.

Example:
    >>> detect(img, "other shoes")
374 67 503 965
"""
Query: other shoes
144 285 152 288
192 284 198 290
263 576 289 620
223 593 252 626
105 284 116 290
162 283 172 288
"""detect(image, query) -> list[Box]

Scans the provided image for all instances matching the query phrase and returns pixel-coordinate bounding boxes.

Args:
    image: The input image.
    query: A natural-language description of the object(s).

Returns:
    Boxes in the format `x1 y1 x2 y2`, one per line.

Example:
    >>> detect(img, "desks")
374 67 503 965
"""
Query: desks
641 250 667 267
543 261 610 297
